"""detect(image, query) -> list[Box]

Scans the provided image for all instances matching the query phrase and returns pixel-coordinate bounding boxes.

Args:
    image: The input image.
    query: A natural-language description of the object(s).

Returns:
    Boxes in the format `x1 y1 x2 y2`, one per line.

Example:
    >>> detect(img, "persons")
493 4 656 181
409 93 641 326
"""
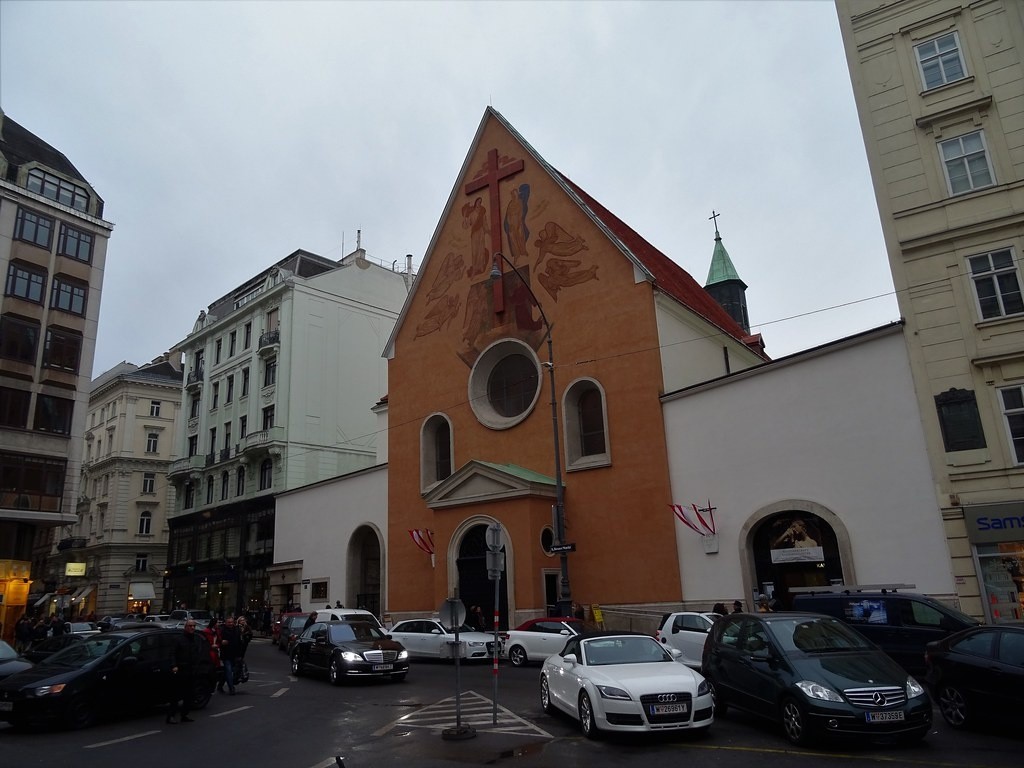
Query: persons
773 519 827 585
167 615 253 725
469 606 485 633
572 601 584 621
713 601 745 614
260 606 275 637
325 600 344 609
303 611 319 632
15 613 66 654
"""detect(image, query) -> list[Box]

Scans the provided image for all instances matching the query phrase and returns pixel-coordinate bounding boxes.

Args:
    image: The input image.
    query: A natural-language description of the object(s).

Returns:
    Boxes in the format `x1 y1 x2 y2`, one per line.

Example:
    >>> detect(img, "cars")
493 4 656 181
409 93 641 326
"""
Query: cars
924 622 1024 739
539 630 715 739
272 607 389 653
654 612 725 672
701 612 933 748
289 621 410 686
503 617 601 667
0 608 224 730
390 618 504 664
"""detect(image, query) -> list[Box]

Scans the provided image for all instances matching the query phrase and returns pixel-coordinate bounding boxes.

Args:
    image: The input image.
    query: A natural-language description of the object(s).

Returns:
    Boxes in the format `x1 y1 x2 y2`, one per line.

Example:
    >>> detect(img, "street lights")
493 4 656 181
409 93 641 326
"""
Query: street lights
490 251 577 614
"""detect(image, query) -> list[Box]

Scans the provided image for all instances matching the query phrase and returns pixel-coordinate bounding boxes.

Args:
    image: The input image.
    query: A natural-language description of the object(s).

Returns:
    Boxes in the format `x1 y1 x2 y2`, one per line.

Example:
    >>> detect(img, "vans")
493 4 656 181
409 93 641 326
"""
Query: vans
788 584 986 684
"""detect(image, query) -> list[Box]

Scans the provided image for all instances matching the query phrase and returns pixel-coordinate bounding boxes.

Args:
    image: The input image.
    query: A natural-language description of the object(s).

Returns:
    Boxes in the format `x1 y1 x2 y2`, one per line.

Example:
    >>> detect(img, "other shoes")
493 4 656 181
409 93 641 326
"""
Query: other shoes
229 687 235 695
217 687 225 693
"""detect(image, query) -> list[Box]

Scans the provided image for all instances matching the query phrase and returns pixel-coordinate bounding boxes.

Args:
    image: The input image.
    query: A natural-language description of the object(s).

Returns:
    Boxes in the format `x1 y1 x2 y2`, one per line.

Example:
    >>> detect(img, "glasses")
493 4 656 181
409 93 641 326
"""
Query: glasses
186 625 196 628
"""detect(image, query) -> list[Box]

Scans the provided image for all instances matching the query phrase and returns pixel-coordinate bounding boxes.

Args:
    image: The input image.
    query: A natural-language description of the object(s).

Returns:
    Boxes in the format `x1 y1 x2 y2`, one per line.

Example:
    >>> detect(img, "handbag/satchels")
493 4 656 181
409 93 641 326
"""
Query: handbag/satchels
232 663 241 685
238 660 249 683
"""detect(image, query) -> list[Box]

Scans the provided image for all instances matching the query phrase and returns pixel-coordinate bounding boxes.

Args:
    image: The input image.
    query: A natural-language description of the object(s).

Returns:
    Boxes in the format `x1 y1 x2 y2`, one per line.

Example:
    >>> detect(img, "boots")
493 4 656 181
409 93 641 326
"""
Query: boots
165 698 178 724
179 702 195 722
